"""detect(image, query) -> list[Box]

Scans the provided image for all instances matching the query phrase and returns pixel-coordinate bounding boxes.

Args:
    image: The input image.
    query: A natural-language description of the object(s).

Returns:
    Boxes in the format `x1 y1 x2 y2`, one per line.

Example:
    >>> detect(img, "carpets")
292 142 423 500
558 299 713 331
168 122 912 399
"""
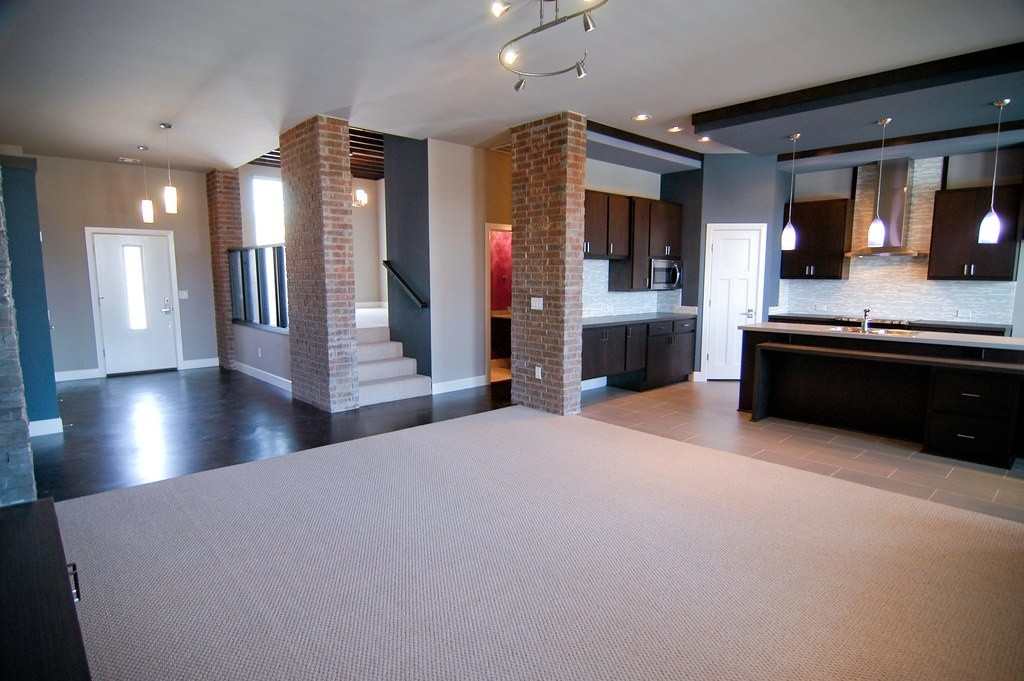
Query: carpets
53 406 1024 681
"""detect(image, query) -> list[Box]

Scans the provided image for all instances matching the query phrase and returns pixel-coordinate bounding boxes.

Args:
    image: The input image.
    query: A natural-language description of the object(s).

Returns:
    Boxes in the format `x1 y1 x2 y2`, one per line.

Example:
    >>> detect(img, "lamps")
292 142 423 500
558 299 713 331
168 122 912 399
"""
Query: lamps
980 98 1013 244
781 133 802 250
137 145 154 223
867 118 892 248
353 188 369 207
490 0 609 92
160 124 178 213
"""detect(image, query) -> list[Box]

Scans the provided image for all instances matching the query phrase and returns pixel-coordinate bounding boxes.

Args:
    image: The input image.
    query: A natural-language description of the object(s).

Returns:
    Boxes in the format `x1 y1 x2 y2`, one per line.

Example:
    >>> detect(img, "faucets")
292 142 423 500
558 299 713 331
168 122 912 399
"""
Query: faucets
861 307 871 333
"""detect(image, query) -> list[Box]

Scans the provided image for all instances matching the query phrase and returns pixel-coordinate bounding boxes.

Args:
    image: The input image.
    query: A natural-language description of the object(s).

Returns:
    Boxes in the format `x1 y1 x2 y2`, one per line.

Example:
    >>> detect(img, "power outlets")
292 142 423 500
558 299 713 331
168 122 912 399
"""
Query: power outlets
258 348 261 359
958 309 973 319
815 304 826 311
535 367 541 380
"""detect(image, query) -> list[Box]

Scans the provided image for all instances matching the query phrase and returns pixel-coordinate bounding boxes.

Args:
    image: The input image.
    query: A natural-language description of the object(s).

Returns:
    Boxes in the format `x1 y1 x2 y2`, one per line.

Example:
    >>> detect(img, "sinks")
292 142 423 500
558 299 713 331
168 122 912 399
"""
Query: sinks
876 330 921 337
830 326 876 333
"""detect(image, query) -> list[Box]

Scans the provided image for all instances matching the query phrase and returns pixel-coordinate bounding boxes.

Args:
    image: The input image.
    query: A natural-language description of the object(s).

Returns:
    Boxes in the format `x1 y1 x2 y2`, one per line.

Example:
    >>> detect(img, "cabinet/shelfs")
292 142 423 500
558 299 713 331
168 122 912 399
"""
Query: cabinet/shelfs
584 193 608 255
648 319 698 381
623 325 649 371
927 185 1024 282
582 327 628 378
926 369 1017 470
609 195 648 292
650 201 684 256
783 198 856 280
607 194 630 256
